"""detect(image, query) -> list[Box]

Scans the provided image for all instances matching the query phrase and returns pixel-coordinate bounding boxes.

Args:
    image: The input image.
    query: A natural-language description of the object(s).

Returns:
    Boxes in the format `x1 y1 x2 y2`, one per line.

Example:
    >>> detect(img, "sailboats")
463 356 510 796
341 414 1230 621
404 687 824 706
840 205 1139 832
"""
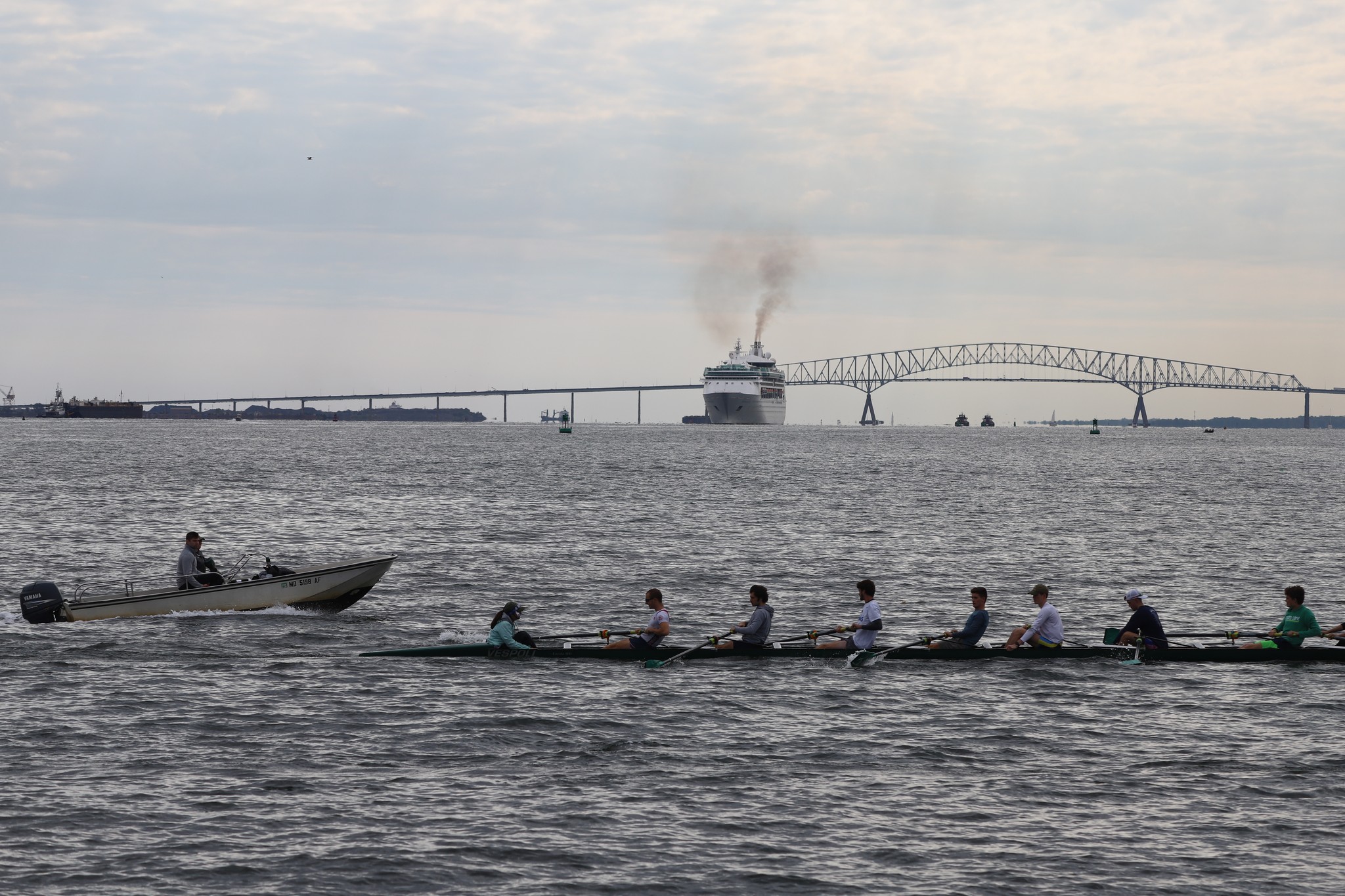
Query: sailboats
1328 408 1333 429
1049 410 1057 426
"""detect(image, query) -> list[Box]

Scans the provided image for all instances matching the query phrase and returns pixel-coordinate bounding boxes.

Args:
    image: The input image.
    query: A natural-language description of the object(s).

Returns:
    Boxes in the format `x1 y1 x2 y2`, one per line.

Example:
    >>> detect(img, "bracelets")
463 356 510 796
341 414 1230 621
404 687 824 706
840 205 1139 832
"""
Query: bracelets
1272 629 1276 632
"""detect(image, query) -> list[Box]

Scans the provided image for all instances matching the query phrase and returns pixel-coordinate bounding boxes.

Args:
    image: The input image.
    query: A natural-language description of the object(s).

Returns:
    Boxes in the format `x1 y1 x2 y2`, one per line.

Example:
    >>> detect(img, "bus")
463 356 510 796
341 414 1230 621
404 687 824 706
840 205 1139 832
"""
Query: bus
1297 387 1311 389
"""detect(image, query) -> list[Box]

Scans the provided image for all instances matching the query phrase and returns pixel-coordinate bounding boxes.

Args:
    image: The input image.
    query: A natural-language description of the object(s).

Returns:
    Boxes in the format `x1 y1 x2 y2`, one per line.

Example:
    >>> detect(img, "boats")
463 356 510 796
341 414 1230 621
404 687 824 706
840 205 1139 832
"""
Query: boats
955 412 970 426
36 389 145 419
1203 429 1214 433
333 413 339 422
46 384 65 415
837 420 842 425
682 405 712 425
235 414 243 421
558 414 573 433
361 644 1345 663
700 338 787 425
981 414 995 427
19 553 398 624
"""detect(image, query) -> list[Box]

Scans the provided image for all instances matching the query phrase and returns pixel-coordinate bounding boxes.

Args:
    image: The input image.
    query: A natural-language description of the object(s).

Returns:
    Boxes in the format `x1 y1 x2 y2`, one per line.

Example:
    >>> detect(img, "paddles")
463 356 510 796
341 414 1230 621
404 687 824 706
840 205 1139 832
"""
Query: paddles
852 630 955 670
766 625 857 647
1318 631 1345 642
1122 630 1142 666
1102 627 1298 645
527 624 648 641
644 624 744 670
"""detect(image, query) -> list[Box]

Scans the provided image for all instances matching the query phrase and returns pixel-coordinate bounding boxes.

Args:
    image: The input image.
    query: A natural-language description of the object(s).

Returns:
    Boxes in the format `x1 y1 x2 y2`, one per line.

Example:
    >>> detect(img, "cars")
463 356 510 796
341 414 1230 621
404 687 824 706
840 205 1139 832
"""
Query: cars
963 377 970 379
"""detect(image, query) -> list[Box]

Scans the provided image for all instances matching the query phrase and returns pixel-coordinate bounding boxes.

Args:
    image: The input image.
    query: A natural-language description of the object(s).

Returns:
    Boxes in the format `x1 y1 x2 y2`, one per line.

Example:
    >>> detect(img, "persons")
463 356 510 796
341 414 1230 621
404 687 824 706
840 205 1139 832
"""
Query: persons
712 585 774 650
924 587 989 650
487 602 538 650
1003 584 1063 651
815 579 883 649
1237 585 1321 649
176 531 225 590
1319 622 1345 640
1112 589 1168 649
600 588 670 649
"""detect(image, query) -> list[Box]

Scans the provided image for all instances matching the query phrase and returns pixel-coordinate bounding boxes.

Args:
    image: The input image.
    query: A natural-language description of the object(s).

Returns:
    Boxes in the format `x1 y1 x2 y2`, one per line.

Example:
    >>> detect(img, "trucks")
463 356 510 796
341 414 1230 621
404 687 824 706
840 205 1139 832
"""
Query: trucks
1271 385 1280 387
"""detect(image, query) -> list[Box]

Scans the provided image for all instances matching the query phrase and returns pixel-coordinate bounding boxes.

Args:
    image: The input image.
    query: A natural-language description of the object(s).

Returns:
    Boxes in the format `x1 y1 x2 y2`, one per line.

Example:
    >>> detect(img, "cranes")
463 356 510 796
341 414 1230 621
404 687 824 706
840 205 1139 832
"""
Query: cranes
0 384 16 405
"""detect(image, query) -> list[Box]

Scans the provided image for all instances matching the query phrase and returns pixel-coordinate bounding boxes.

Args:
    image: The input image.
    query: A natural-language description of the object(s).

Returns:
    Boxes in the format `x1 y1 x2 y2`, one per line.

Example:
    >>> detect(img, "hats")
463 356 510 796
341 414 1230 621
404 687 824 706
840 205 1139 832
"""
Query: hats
1027 584 1049 595
1124 589 1148 600
199 535 205 541
503 602 524 614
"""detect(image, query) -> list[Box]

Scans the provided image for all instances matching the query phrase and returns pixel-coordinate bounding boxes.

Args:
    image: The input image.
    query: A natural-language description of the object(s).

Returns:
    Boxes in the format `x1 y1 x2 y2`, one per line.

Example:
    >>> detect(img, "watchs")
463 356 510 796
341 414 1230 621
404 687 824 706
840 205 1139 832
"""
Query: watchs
1014 642 1020 646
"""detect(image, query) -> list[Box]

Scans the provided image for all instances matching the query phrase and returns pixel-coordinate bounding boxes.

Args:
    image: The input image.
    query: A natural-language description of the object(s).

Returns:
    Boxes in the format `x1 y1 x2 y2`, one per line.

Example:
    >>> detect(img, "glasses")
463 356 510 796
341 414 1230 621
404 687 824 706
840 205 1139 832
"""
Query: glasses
645 597 654 601
